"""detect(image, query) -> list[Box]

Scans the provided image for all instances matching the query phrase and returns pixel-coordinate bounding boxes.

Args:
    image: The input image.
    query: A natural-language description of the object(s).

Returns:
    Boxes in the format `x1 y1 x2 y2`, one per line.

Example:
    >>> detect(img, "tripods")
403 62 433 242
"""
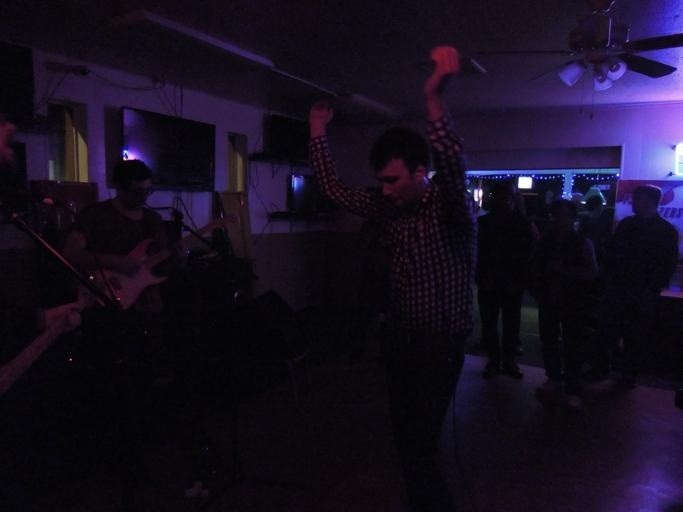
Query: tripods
196 228 304 511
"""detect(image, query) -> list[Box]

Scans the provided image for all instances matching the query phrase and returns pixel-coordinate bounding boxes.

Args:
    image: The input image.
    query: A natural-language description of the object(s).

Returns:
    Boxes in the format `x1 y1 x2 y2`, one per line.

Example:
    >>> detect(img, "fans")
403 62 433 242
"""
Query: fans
471 0 683 81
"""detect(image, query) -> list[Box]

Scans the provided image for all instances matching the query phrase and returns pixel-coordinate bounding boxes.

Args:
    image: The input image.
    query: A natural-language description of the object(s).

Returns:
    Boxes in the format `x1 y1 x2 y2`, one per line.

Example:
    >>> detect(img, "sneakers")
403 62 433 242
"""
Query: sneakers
479 358 643 409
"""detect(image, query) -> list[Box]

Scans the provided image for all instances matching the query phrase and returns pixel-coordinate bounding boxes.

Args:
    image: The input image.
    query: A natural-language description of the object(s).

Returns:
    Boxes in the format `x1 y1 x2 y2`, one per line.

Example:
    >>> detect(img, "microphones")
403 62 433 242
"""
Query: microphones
0 202 31 231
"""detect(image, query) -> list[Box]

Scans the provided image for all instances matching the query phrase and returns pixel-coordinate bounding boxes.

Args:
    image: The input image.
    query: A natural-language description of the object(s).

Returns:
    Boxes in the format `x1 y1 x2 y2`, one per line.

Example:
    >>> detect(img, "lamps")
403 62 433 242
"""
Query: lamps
556 54 627 92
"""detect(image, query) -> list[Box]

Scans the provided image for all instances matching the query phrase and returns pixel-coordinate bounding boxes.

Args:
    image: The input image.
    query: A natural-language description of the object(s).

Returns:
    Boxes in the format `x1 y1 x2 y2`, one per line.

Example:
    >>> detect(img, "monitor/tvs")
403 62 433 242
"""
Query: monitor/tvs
120 105 216 192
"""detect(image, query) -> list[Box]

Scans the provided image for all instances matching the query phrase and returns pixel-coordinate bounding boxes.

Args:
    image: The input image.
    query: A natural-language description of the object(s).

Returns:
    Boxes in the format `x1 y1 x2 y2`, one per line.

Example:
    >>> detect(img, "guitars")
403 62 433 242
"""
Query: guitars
91 214 240 310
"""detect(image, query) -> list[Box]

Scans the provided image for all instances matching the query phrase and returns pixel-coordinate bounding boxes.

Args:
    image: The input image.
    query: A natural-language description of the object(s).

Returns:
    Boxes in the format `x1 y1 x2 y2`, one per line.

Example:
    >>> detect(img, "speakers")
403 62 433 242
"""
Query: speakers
273 113 310 160
0 41 33 120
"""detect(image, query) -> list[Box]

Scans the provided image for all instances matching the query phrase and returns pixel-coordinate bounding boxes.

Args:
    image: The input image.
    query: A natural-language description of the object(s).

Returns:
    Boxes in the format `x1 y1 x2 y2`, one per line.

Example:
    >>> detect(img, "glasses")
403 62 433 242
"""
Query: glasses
121 185 155 197
